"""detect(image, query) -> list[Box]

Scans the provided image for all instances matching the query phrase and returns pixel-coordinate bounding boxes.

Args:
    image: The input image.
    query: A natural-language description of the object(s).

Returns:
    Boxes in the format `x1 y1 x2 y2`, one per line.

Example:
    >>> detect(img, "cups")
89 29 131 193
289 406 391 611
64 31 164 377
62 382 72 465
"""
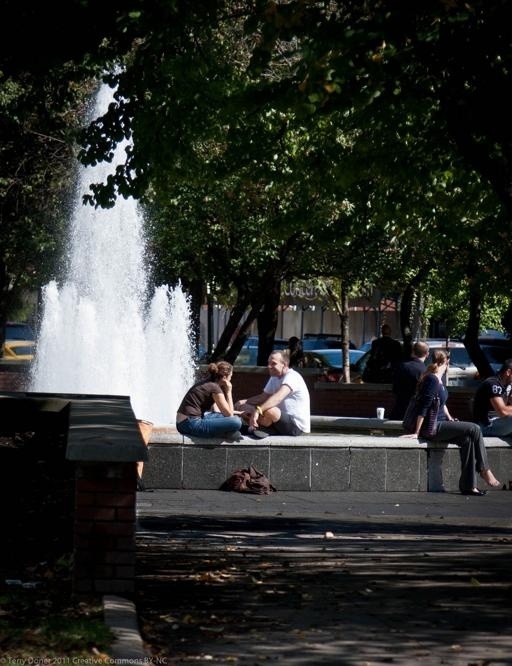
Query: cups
377 407 385 420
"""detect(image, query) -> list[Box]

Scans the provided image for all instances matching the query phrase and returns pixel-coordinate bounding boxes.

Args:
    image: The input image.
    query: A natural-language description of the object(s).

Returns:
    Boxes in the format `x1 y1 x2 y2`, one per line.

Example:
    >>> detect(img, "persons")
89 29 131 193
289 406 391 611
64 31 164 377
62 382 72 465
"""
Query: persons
361 324 512 495
176 336 311 439
135 419 154 491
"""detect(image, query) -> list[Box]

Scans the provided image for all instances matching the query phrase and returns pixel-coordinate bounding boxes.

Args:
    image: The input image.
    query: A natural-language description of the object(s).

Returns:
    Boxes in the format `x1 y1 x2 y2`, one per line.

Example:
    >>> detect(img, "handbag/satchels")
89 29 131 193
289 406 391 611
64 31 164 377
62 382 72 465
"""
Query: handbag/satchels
402 396 439 438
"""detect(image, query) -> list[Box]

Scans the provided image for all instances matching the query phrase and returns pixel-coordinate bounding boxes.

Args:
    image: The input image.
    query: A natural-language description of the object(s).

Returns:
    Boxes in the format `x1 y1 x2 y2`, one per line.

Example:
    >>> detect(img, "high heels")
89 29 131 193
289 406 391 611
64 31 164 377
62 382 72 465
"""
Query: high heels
461 486 487 497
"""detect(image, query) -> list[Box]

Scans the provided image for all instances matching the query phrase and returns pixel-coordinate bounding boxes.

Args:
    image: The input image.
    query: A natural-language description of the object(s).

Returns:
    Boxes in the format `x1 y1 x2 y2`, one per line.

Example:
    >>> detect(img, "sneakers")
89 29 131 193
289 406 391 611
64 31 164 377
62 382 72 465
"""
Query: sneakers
223 430 241 439
253 429 269 439
